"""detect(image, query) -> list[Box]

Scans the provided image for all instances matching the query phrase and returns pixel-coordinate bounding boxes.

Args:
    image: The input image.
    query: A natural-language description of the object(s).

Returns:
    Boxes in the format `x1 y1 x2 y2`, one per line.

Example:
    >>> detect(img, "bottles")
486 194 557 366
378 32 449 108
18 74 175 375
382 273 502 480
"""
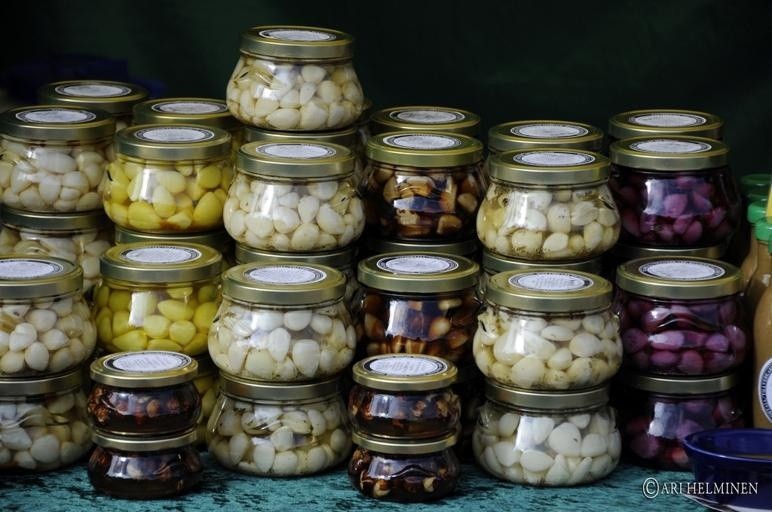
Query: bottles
0 29 740 502
740 170 772 428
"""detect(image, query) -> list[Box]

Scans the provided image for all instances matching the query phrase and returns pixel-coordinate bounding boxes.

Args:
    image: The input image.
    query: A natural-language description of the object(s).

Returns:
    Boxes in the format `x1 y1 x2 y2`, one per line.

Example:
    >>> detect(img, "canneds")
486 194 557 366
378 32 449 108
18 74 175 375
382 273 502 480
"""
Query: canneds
0 24 747 502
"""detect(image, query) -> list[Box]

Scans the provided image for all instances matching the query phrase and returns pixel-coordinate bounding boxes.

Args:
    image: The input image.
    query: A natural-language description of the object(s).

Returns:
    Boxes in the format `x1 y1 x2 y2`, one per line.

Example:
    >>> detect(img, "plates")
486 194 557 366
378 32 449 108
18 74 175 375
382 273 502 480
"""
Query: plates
679 482 772 512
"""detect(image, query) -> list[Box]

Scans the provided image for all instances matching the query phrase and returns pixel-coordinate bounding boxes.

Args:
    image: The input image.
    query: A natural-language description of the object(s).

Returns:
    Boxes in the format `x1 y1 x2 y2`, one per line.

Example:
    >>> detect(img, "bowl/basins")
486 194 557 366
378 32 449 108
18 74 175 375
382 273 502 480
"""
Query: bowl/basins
682 428 771 509
